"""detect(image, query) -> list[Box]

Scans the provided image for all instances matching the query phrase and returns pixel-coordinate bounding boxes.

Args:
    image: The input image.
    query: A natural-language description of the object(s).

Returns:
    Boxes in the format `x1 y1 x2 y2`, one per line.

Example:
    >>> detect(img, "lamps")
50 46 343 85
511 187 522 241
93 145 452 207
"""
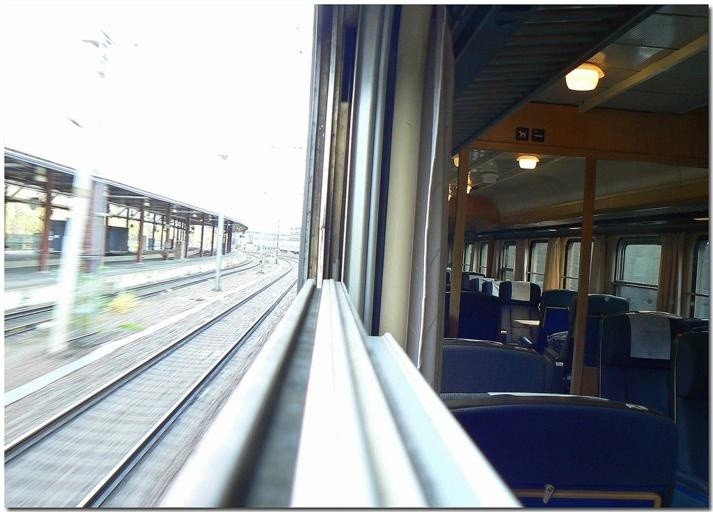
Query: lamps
564 62 607 92
516 154 541 170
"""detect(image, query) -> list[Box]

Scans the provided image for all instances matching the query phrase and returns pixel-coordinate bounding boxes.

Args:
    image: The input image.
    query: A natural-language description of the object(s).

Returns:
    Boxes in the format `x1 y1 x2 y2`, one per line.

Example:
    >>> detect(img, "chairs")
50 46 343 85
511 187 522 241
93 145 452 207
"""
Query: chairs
441 266 708 508
